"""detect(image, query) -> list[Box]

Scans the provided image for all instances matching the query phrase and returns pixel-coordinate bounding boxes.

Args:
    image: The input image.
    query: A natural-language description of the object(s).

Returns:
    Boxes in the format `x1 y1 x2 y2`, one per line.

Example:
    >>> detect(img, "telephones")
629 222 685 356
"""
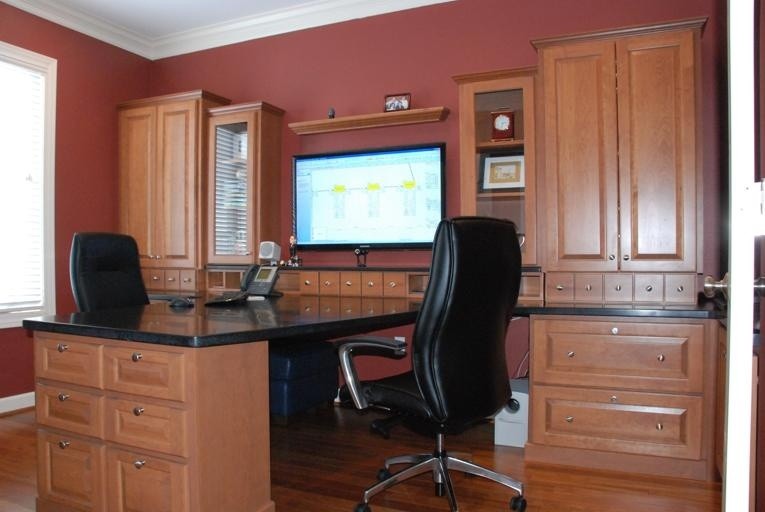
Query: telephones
239 264 283 297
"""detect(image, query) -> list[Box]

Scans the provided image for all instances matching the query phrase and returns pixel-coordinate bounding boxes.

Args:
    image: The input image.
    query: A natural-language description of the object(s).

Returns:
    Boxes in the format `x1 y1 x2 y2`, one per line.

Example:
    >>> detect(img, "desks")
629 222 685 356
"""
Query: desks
21 297 424 510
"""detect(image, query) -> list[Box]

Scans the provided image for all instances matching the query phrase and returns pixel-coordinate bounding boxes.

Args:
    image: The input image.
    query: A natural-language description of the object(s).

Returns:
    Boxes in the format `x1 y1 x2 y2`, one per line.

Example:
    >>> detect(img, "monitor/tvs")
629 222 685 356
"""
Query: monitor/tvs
290 142 447 252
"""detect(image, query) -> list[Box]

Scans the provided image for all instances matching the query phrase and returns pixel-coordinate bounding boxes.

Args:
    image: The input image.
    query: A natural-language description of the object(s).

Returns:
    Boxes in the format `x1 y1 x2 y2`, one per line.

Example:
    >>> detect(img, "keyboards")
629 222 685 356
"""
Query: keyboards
205 292 248 307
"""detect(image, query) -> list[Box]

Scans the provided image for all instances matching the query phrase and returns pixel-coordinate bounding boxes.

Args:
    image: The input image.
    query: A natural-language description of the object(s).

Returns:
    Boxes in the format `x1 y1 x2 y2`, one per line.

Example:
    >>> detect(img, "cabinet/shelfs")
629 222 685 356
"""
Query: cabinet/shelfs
529 16 710 310
115 88 228 296
278 269 543 303
206 101 287 265
528 317 718 482
450 65 537 267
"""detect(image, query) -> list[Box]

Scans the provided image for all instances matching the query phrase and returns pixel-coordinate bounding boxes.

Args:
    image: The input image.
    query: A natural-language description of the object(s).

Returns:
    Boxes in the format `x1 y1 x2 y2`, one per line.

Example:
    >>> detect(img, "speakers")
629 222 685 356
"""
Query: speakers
258 241 282 263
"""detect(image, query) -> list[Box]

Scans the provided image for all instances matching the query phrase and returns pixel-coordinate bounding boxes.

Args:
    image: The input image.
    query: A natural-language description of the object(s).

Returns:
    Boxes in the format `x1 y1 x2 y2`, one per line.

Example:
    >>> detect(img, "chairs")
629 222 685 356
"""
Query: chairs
69 231 149 315
339 216 527 509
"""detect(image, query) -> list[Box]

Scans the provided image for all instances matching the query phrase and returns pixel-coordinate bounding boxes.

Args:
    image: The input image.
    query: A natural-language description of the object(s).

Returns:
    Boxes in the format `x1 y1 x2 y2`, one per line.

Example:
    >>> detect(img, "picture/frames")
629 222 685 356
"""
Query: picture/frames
383 93 411 111
479 149 524 190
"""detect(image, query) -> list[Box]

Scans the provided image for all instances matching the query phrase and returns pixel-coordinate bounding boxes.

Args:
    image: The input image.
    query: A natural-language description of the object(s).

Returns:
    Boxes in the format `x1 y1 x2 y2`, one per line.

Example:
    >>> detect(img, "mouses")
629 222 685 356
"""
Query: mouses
170 297 194 308
